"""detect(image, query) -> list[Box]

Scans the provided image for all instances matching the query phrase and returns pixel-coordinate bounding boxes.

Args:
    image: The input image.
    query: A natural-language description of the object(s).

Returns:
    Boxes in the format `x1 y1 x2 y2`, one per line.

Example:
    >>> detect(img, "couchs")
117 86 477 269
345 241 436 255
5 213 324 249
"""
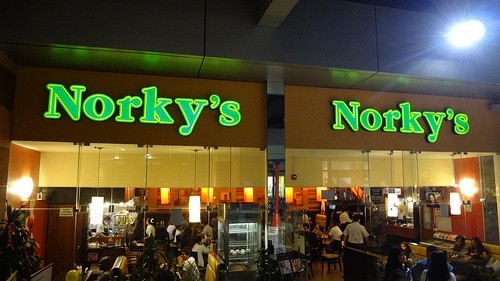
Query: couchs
110 255 129 281
204 251 225 281
410 245 427 261
463 240 500 262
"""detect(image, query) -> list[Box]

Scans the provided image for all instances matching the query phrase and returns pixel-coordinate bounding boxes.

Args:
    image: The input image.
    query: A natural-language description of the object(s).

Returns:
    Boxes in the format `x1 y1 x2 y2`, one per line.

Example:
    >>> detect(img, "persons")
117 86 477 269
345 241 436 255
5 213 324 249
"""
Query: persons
66 197 370 281
385 241 456 281
427 193 437 203
450 235 488 276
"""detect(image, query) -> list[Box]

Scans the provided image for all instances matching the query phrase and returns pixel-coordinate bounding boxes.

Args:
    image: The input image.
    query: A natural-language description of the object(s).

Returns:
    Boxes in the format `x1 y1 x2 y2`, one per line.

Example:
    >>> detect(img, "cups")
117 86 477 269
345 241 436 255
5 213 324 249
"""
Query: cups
230 249 246 255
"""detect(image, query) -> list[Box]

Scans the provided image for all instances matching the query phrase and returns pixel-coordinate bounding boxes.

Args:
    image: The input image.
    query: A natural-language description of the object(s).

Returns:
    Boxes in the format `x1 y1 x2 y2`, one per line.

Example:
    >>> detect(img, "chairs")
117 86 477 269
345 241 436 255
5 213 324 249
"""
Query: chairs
320 242 343 274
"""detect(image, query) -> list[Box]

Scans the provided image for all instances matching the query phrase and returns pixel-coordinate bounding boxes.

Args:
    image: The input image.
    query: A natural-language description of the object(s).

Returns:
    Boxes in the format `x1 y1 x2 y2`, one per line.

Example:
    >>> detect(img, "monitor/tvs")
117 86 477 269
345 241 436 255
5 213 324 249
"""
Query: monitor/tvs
29 263 53 281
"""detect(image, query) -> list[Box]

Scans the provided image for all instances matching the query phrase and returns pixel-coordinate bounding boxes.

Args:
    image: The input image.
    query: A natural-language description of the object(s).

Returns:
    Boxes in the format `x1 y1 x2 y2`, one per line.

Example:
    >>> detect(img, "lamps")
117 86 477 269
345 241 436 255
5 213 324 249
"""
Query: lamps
449 192 461 215
202 147 214 203
90 148 105 225
244 187 254 203
387 153 398 217
160 187 170 205
316 187 327 201
188 151 201 223
285 187 294 203
